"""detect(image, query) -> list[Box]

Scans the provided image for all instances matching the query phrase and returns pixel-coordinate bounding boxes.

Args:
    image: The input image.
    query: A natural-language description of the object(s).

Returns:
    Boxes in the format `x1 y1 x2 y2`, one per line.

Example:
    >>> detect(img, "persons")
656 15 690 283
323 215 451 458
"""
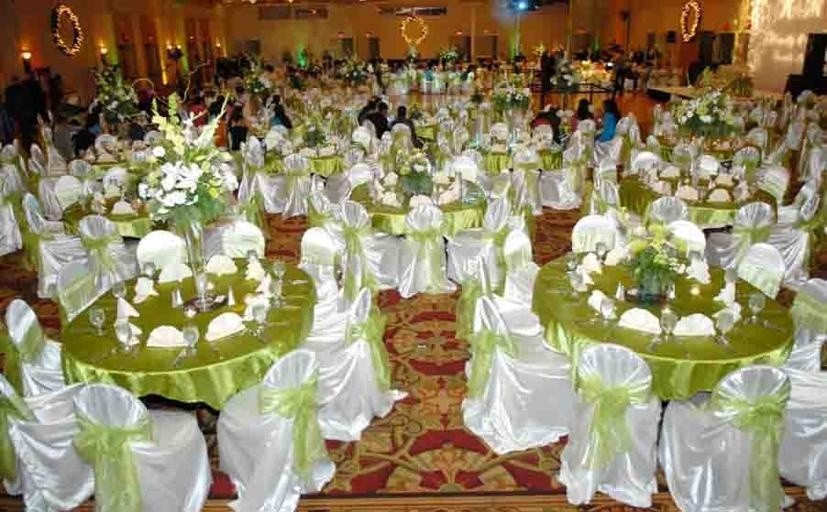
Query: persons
86 49 292 150
395 57 526 80
598 99 621 142
539 40 666 109
299 47 348 79
530 111 556 134
357 95 423 150
570 98 598 132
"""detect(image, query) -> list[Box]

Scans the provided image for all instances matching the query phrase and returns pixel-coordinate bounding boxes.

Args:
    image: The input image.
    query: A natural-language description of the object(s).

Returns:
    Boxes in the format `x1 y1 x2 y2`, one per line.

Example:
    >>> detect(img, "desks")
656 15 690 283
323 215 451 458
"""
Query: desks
60 257 317 413
532 250 796 403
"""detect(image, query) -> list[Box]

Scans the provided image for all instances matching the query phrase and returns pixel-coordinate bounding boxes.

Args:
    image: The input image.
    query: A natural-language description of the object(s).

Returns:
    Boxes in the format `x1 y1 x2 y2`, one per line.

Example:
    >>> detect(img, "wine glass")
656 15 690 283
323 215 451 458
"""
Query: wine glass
564 243 765 345
83 247 287 354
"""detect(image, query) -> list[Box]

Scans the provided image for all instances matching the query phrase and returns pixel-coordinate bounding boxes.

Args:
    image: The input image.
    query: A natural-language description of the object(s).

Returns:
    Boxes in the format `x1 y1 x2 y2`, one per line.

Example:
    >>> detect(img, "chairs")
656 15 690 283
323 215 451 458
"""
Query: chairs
660 219 707 262
220 218 270 261
555 343 661 507
2 295 66 399
459 295 573 456
213 346 339 511
55 259 101 328
655 364 791 512
296 225 340 297
569 215 619 256
2 371 94 512
305 283 410 442
134 230 190 271
71 385 211 510
736 242 785 299
305 251 365 320
2 68 827 296
771 366 827 502
500 229 543 303
473 256 541 335
783 275 827 370
